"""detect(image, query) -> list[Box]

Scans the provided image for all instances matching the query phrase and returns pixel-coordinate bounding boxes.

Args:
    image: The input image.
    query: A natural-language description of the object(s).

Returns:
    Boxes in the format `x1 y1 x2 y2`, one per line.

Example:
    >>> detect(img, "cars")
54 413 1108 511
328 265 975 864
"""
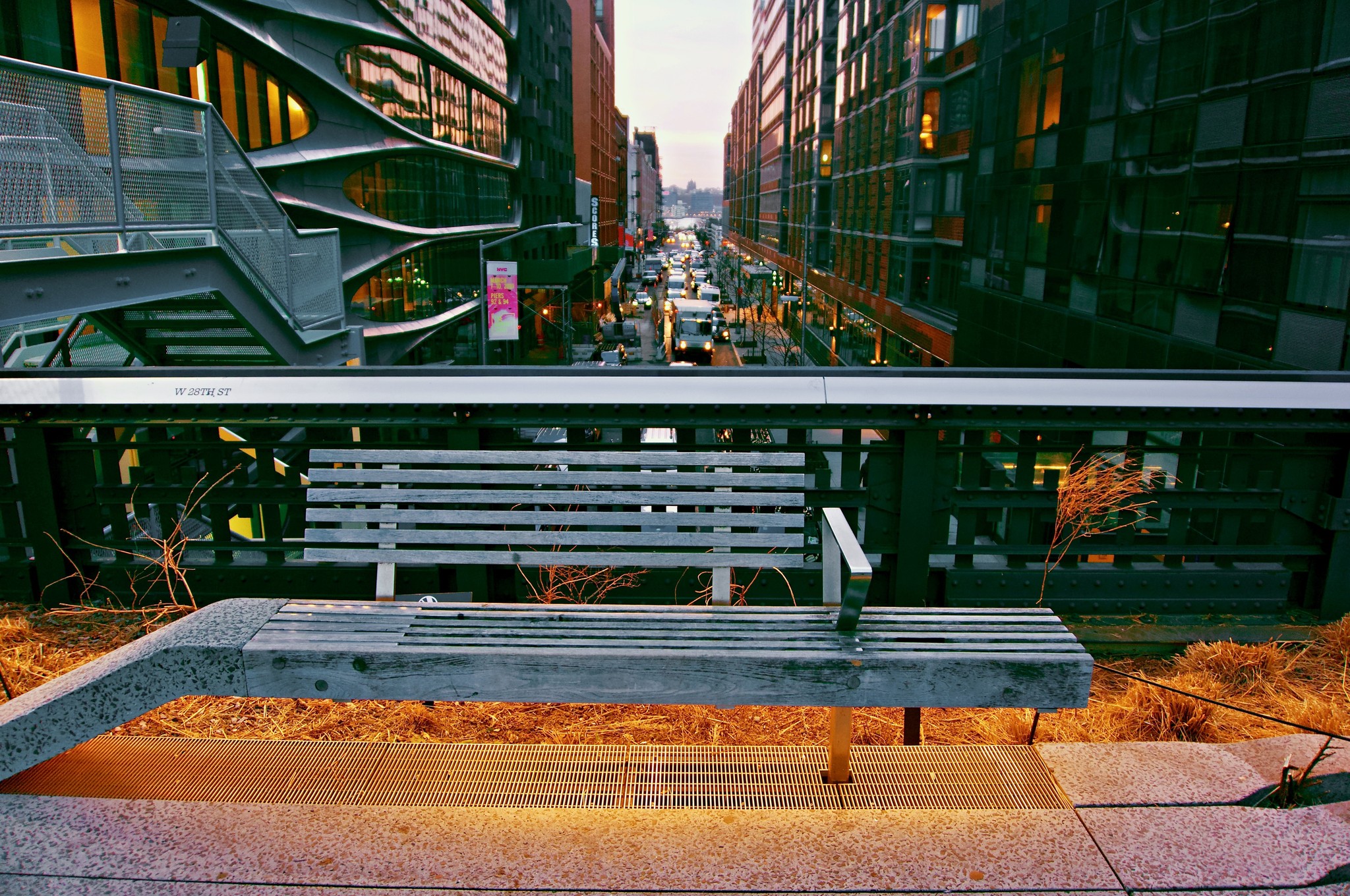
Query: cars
669 362 697 367
663 250 716 299
643 248 668 270
711 305 730 341
667 230 701 251
634 292 651 309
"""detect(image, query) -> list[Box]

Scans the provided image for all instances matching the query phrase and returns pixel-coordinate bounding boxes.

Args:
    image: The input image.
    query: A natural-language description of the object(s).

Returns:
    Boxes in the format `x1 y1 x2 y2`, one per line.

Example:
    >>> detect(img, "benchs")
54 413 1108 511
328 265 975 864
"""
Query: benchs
0 449 1093 784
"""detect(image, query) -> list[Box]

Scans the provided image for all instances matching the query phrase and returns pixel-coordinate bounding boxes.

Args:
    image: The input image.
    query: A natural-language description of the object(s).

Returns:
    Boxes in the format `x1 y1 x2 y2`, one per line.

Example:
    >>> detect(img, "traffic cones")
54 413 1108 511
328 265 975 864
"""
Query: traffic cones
649 265 652 270
643 285 648 293
654 282 657 288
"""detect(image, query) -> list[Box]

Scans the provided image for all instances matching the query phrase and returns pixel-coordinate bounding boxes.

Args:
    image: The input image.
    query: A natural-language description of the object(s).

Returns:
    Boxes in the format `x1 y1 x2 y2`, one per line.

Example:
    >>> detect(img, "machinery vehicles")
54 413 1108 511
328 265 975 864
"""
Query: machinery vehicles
602 258 642 361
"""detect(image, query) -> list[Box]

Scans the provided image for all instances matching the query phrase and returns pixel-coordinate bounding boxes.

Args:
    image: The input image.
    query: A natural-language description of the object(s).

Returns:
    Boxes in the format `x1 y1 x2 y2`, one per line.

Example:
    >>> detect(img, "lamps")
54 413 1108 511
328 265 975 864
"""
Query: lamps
161 15 215 67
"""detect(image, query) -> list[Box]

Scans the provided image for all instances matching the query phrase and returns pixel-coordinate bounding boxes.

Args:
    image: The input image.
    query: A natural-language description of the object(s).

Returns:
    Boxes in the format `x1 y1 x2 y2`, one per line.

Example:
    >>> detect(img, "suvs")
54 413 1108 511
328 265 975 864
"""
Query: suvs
640 428 677 452
584 343 627 366
642 271 658 286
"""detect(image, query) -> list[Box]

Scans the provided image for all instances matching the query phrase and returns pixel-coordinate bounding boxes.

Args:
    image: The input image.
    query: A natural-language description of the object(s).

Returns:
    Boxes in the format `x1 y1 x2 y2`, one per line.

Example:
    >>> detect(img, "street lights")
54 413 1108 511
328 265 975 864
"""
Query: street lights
780 214 807 365
479 223 583 366
703 220 741 335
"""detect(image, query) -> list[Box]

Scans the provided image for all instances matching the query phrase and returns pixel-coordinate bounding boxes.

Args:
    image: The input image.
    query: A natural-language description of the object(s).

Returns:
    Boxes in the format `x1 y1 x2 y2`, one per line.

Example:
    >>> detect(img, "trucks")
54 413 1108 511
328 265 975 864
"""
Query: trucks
672 298 714 362
643 257 663 276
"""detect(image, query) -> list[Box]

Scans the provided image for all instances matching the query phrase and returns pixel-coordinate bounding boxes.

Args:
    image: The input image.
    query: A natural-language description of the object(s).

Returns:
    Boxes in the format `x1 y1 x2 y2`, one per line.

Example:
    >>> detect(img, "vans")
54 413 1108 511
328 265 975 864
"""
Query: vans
697 284 720 306
